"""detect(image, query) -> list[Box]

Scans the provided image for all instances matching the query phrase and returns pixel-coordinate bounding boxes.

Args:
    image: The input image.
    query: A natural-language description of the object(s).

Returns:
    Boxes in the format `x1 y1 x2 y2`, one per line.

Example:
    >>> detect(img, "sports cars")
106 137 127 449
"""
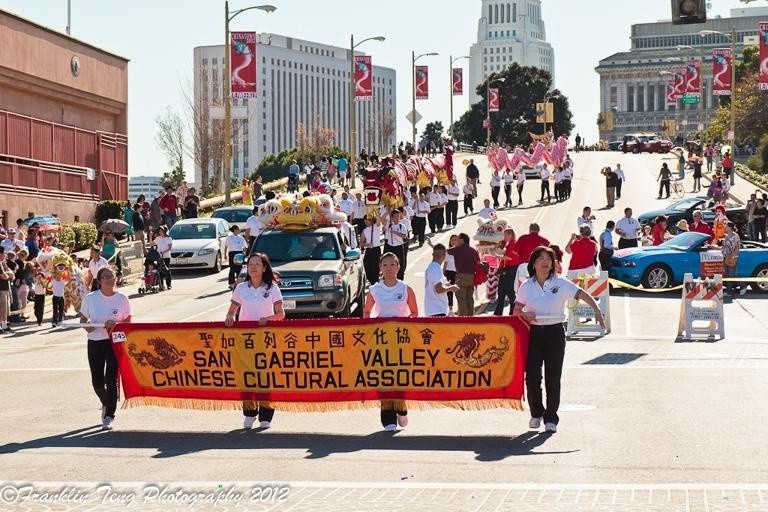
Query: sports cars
607 229 768 300
633 193 750 240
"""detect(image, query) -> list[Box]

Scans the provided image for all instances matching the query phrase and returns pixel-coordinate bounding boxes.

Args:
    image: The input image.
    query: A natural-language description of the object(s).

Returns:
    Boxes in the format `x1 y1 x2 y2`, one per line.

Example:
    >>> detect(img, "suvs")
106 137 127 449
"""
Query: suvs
228 210 369 320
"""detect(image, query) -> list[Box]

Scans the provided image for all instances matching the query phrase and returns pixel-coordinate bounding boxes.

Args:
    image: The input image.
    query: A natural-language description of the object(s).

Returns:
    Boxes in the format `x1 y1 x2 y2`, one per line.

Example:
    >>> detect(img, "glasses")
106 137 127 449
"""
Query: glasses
99 274 115 279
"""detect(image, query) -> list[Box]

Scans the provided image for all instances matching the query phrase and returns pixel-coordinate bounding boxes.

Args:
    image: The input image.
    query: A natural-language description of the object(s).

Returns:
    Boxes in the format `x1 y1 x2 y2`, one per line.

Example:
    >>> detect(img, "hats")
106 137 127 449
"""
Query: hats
159 225 168 234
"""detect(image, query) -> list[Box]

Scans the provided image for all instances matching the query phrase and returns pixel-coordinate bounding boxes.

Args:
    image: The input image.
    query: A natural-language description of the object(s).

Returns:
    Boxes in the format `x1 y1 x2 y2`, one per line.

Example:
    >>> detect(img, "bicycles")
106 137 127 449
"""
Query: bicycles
665 175 686 198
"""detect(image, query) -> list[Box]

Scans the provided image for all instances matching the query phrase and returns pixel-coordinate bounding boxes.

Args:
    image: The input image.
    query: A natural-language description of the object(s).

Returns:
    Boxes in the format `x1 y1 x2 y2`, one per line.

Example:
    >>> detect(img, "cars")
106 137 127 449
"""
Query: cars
167 215 232 274
513 153 554 180
208 205 255 245
607 133 675 155
251 194 266 209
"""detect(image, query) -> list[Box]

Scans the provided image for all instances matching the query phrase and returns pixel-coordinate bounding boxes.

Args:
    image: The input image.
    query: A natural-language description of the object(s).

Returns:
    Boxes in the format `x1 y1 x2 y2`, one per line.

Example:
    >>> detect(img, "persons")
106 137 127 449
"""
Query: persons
0 131 768 433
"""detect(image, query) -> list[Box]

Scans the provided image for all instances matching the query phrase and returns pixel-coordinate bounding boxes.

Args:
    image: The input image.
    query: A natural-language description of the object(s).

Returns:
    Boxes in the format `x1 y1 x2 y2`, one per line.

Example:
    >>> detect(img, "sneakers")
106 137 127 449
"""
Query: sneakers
384 424 398 432
243 415 257 429
528 407 545 429
101 404 107 422
259 420 271 429
102 415 113 430
544 421 558 434
397 414 408 427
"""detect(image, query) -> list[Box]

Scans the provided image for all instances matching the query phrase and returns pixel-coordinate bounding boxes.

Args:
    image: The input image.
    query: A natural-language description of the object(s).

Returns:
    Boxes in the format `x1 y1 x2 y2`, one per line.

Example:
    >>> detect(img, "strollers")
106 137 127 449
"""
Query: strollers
136 246 165 296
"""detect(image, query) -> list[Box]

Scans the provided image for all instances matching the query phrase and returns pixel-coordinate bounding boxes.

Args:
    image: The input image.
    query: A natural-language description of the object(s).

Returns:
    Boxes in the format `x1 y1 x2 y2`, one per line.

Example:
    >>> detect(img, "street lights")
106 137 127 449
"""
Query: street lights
348 34 388 190
412 51 441 146
487 77 507 147
605 103 620 139
223 4 279 207
543 94 561 134
658 26 741 186
448 54 473 138
